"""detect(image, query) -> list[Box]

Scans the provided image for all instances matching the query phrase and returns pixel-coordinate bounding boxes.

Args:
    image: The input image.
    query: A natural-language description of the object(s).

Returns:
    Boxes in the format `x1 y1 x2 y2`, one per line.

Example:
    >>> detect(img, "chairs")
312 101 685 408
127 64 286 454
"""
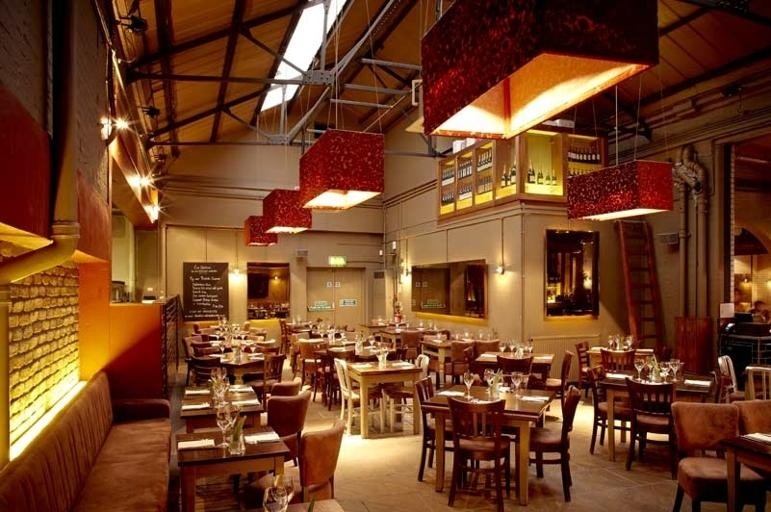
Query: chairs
179 322 313 469
669 401 768 512
732 400 771 434
414 375 583 512
278 317 574 438
245 419 345 505
574 339 771 470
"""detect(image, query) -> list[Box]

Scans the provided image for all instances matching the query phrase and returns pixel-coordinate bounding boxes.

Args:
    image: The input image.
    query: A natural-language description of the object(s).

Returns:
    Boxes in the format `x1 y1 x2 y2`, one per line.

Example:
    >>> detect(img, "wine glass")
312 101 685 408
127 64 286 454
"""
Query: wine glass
214 321 257 360
460 366 523 402
633 355 682 383
306 320 374 352
208 364 234 450
392 316 433 332
452 327 493 341
605 333 634 351
495 337 533 358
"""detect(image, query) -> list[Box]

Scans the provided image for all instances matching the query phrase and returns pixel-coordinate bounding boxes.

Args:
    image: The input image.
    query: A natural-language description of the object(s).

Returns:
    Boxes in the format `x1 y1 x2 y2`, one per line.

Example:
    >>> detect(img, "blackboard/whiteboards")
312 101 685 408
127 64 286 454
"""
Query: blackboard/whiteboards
183 262 229 322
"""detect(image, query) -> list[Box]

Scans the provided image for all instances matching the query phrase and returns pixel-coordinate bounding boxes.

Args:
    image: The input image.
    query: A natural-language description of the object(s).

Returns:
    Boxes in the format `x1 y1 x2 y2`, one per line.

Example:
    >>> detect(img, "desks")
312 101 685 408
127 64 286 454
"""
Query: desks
718 433 771 512
174 425 291 511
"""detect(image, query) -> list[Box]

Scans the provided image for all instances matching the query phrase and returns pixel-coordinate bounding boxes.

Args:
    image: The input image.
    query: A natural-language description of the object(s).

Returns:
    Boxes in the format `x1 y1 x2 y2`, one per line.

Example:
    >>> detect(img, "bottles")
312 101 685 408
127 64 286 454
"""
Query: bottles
123 291 133 302
566 145 600 178
501 154 557 190
438 148 494 206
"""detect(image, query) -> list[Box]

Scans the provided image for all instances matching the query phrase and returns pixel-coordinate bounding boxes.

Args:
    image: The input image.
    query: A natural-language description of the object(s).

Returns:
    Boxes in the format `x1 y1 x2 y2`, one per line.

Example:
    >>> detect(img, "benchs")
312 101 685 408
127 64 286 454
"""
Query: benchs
1 368 173 511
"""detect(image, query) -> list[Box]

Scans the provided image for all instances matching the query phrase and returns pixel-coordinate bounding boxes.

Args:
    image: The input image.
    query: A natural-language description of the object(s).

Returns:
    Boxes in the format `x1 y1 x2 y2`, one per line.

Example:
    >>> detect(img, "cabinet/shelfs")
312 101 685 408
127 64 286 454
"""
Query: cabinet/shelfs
429 125 611 228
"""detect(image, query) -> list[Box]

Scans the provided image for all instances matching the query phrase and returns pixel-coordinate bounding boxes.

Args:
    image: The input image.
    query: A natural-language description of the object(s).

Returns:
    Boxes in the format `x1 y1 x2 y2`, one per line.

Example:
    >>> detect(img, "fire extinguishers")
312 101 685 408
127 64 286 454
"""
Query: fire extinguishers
394 310 402 323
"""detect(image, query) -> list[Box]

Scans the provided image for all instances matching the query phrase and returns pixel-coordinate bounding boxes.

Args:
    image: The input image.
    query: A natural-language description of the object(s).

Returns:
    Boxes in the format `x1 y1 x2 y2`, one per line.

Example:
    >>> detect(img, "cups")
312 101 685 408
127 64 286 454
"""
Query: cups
261 470 295 512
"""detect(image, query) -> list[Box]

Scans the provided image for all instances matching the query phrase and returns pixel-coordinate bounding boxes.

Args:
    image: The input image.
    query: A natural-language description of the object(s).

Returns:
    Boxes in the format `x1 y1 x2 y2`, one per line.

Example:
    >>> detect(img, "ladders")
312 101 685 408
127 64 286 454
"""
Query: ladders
613 219 670 363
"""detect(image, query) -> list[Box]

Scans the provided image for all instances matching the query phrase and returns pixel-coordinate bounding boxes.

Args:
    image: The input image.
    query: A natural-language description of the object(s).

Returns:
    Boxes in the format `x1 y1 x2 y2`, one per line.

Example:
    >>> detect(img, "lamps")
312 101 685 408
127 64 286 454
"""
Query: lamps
560 69 681 228
295 1 388 216
260 80 315 237
243 108 281 250
420 3 662 142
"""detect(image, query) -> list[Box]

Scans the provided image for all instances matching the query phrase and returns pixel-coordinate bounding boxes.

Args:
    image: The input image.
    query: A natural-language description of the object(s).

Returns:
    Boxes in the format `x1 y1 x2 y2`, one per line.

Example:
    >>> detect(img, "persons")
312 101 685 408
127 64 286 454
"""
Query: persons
747 301 771 322
735 287 745 311
